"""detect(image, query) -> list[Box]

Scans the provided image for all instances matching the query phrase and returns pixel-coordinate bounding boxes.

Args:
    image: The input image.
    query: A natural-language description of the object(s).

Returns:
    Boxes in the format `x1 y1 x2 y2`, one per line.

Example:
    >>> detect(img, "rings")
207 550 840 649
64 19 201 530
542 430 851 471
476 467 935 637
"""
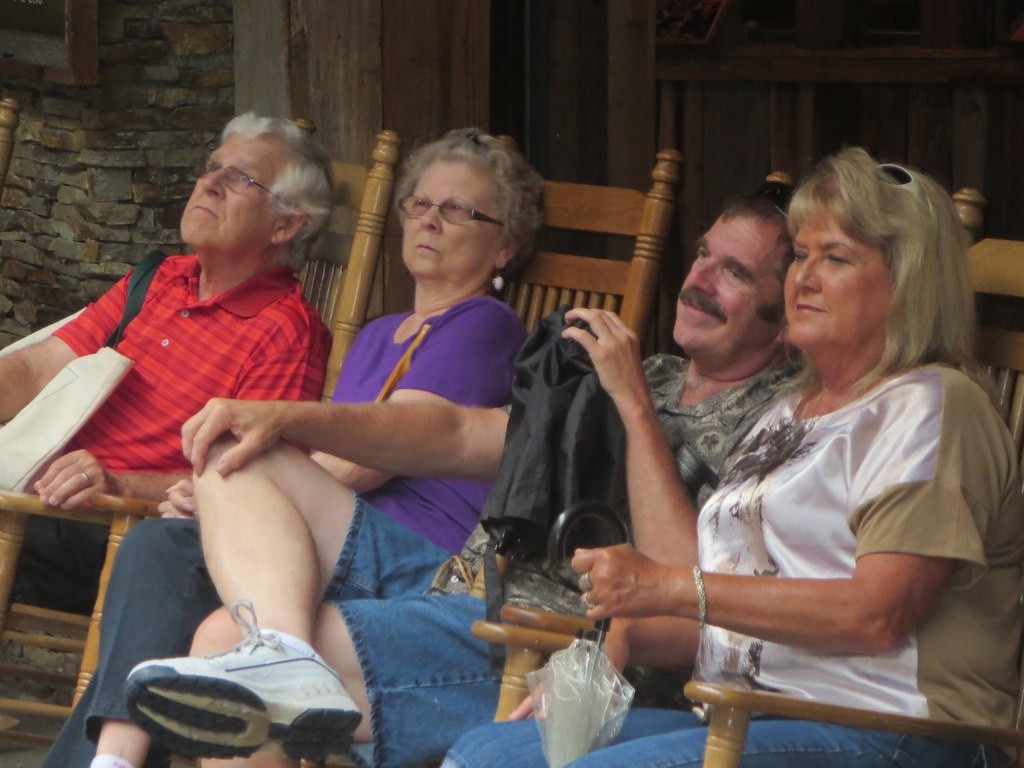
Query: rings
581 593 595 609
81 472 91 486
580 573 593 591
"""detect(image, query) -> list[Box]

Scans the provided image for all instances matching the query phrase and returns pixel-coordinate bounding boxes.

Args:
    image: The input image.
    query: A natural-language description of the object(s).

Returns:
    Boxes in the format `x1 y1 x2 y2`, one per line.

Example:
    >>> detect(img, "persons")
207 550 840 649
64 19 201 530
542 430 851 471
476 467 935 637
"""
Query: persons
442 144 1022 768
41 128 552 768
117 185 810 768
0 111 340 619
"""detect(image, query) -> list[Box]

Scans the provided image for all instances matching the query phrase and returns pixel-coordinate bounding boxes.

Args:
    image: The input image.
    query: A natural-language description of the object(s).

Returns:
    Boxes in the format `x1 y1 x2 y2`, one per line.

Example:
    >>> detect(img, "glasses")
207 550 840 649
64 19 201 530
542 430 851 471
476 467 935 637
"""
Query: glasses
193 155 299 209
398 194 504 228
877 163 916 196
755 179 795 219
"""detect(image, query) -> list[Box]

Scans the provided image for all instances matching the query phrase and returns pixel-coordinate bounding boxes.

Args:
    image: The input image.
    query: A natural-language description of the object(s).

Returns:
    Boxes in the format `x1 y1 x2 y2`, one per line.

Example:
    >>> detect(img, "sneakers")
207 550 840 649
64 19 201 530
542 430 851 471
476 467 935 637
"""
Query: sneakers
124 597 363 759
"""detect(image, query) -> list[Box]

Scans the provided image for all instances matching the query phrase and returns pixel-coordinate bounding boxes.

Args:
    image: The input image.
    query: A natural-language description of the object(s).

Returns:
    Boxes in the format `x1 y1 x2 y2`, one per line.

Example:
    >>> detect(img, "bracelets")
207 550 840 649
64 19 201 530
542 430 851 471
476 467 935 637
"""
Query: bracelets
691 566 706 629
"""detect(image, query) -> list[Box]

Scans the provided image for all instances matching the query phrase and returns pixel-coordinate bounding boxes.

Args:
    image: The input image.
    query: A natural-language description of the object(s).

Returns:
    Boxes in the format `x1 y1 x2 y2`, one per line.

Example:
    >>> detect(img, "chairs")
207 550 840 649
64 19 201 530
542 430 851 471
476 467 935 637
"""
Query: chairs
0 116 1024 768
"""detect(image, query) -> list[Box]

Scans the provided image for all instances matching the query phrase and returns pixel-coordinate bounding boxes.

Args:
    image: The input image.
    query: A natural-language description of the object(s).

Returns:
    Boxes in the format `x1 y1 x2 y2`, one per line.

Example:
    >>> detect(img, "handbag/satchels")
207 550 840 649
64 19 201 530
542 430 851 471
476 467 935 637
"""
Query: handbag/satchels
0 249 167 497
